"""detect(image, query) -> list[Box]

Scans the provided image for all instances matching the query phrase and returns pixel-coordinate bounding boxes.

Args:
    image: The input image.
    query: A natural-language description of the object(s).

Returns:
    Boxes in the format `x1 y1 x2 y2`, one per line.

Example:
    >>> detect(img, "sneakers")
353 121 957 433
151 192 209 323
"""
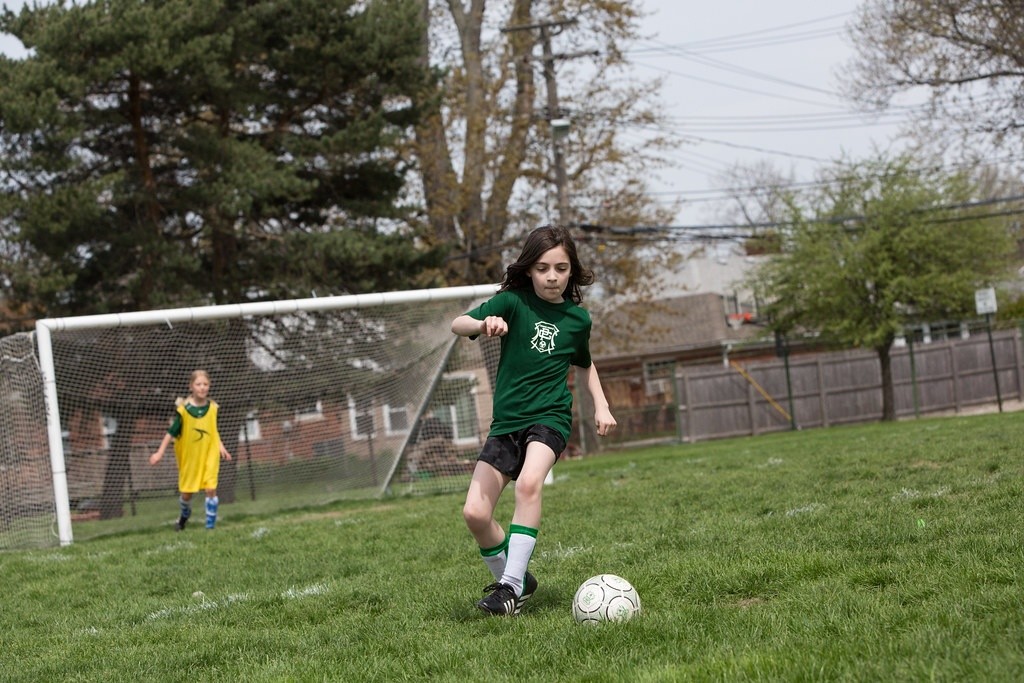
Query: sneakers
478 582 518 617
511 571 538 617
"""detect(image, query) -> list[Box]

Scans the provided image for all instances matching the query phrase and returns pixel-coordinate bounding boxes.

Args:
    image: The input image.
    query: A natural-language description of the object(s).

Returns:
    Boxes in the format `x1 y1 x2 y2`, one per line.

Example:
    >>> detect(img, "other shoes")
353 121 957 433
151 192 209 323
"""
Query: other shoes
175 511 191 531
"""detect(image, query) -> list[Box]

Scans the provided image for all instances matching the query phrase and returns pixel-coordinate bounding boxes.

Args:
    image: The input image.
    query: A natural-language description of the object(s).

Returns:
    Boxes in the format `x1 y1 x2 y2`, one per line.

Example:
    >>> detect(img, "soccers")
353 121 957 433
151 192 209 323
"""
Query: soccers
572 574 641 627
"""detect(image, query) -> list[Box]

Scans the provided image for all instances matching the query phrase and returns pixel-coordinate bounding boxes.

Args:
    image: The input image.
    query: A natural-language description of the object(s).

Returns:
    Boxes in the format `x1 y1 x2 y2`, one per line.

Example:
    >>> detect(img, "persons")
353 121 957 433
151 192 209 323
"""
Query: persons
149 370 232 531
450 224 617 616
